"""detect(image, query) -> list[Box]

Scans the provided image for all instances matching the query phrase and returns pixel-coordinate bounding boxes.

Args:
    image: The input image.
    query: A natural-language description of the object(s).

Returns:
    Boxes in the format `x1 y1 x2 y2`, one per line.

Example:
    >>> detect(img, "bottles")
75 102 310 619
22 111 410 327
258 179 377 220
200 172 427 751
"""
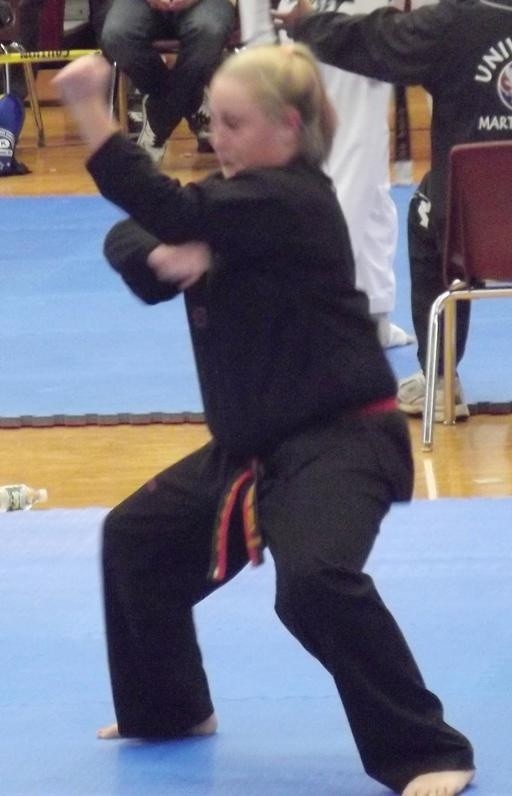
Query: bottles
0 484 49 513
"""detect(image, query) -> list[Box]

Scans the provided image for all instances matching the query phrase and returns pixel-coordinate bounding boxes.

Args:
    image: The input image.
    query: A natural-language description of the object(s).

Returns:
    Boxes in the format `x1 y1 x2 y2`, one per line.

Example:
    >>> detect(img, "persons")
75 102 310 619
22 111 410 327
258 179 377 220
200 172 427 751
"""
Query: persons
49 43 476 796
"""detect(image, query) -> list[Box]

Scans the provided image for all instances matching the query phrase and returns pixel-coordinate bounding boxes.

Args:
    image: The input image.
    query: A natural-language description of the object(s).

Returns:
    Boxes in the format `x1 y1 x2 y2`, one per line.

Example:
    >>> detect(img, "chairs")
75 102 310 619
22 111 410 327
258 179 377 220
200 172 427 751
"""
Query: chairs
114 35 248 140
421 140 511 451
1 0 47 150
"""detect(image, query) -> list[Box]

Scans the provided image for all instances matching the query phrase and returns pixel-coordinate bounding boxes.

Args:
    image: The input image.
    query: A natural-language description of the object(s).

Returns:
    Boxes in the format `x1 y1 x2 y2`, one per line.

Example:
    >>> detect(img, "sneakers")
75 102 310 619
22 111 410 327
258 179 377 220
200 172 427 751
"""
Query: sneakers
395 371 471 424
137 93 167 166
